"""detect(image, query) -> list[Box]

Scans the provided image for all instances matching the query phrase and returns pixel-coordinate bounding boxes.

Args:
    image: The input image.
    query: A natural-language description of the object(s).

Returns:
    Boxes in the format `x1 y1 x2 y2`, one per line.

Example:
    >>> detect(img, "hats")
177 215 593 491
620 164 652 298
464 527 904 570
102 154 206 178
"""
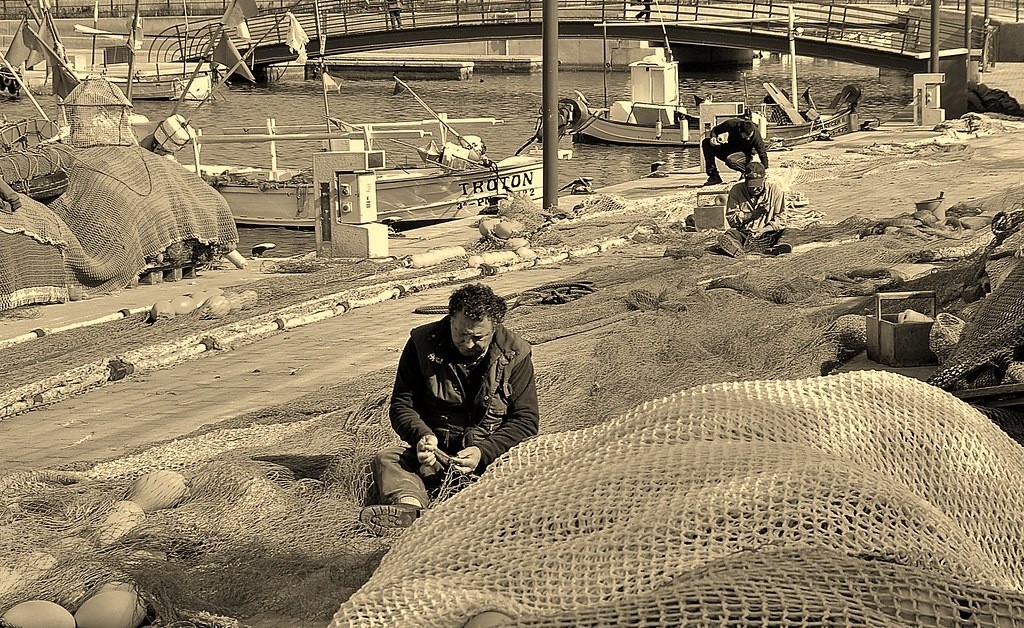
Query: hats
744 162 767 187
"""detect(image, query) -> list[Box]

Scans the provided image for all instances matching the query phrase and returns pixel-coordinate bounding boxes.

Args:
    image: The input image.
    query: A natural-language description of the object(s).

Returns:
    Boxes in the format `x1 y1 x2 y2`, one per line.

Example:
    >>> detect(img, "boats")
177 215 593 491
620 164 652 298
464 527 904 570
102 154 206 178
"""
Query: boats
572 0 862 148
0 0 542 231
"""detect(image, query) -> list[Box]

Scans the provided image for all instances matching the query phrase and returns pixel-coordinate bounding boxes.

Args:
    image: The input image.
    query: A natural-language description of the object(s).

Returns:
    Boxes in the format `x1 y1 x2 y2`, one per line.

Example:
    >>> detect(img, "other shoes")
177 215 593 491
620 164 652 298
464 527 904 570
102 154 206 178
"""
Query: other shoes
739 174 745 181
359 503 432 536
764 243 793 256
717 235 745 258
704 173 723 186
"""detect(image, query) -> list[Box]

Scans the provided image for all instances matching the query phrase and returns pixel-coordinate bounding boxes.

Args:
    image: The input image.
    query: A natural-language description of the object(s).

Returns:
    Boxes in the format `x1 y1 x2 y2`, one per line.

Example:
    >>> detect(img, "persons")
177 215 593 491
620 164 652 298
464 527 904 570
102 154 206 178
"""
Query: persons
635 0 653 22
361 284 539 537
702 118 768 185
718 162 792 258
388 0 403 30
